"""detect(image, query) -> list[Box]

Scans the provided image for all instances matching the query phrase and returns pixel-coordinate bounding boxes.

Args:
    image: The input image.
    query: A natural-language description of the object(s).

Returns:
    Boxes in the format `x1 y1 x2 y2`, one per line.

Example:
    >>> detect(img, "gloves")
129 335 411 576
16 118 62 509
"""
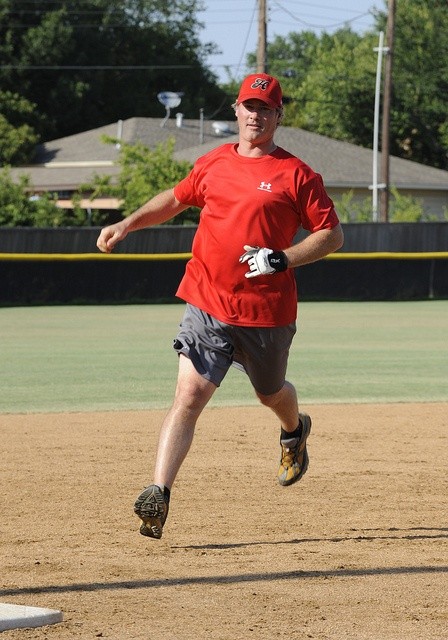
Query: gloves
237 245 288 279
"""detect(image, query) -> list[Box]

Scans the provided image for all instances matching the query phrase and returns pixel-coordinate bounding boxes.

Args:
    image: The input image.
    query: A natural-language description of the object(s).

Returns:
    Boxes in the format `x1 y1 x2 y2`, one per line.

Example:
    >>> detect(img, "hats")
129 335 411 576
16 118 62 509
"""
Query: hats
236 74 283 109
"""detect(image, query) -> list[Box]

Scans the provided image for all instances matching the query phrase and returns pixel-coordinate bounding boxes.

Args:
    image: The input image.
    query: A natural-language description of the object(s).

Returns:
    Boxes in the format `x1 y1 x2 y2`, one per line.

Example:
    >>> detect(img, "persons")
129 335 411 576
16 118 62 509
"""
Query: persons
96 74 345 538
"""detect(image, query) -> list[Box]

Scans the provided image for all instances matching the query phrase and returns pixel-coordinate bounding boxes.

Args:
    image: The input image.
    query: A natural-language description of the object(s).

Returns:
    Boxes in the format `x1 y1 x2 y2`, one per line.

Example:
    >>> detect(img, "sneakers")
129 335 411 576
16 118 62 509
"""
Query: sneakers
134 485 169 539
279 413 312 486
